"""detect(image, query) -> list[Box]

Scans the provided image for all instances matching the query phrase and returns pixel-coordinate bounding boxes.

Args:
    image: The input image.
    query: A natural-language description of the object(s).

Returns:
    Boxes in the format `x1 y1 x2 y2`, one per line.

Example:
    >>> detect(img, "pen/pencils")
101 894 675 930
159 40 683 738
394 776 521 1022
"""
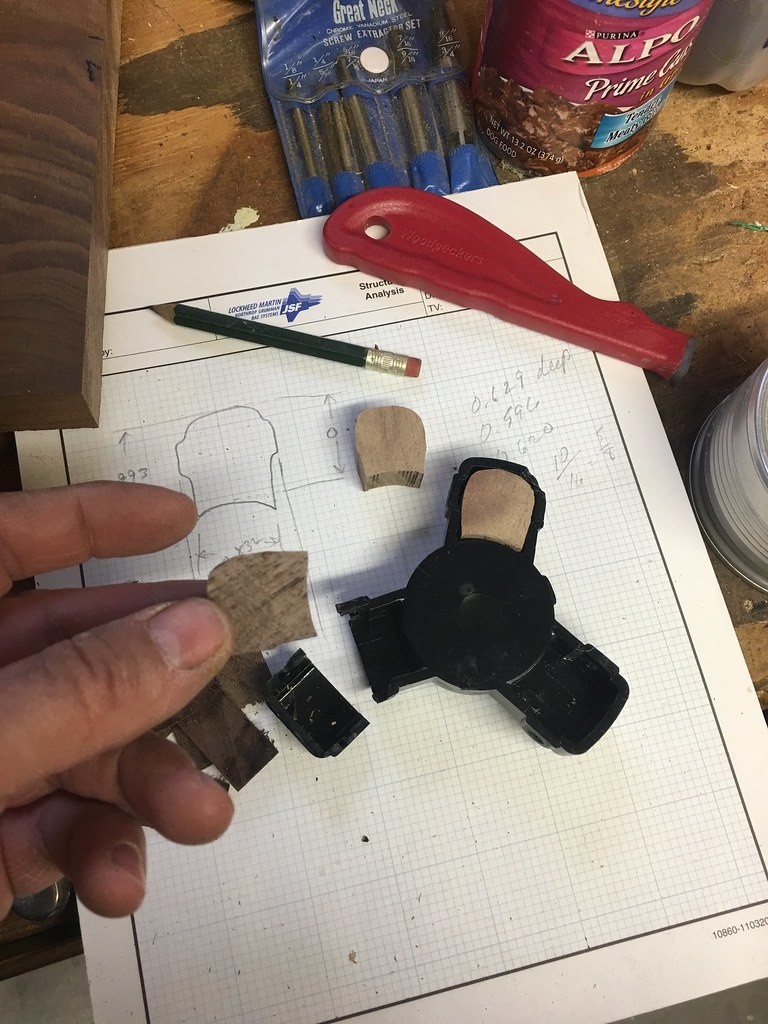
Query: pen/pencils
147 301 423 378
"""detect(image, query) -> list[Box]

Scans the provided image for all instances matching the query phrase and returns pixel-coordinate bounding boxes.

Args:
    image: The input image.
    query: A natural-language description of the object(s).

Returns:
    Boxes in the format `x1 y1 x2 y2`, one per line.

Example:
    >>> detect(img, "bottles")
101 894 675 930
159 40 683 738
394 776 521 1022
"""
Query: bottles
470 0 712 181
689 361 768 593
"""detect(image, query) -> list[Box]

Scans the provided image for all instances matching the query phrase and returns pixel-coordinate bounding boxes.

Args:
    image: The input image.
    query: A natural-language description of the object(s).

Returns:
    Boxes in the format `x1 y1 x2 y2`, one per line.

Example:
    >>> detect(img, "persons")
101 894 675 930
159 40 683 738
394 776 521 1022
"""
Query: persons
1 481 237 929
